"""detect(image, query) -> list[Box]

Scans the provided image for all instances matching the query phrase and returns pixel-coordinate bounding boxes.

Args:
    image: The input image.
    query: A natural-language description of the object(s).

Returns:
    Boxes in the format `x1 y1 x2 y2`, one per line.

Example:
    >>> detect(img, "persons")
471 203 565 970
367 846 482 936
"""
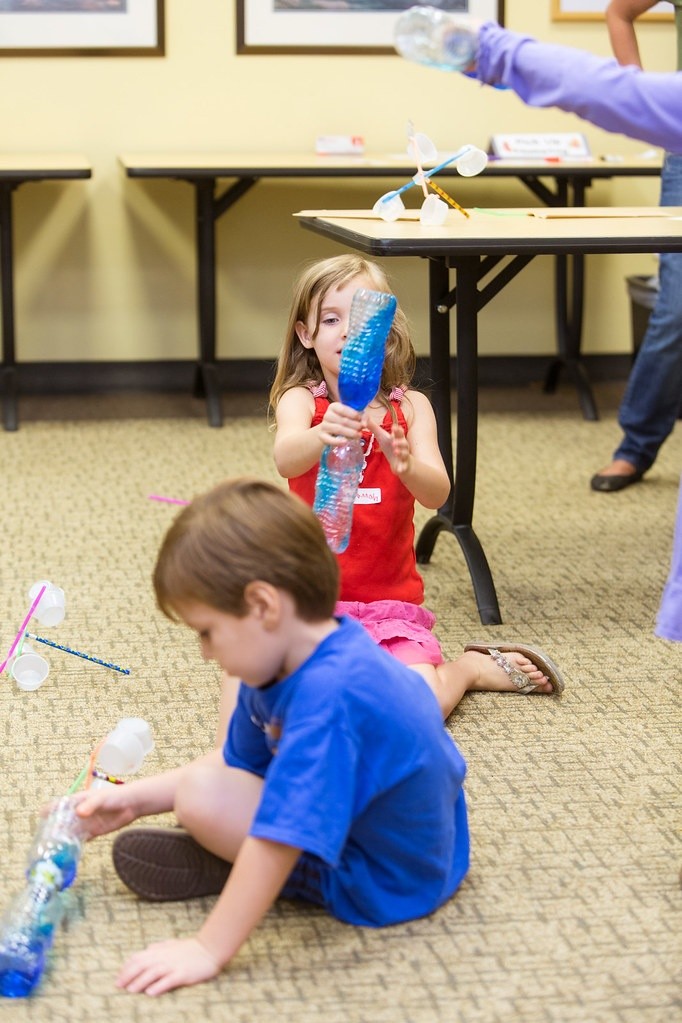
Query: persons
434 15 682 637
589 0 682 491
36 479 473 994
265 254 565 721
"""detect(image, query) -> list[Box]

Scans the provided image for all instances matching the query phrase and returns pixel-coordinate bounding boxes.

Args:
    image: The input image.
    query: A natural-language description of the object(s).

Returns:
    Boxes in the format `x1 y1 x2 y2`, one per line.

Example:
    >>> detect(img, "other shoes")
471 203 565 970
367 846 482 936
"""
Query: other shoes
592 459 643 491
112 826 231 900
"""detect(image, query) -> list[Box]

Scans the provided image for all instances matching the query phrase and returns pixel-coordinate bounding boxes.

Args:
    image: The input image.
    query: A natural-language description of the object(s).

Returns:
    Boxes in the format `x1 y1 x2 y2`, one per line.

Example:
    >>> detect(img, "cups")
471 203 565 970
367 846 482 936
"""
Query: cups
12 655 48 692
372 191 405 222
455 146 488 177
30 582 65 626
419 194 448 228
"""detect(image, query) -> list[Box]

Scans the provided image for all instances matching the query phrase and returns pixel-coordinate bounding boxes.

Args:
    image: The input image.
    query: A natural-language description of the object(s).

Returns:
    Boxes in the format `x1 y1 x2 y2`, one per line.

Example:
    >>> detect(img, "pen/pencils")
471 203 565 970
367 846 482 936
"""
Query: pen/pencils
18 630 131 676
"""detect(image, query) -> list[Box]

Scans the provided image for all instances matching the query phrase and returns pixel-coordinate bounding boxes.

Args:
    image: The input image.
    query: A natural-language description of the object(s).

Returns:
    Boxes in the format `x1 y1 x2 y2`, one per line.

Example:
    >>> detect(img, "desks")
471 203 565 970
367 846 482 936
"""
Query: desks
297 208 682 625
0 157 93 432
116 153 664 430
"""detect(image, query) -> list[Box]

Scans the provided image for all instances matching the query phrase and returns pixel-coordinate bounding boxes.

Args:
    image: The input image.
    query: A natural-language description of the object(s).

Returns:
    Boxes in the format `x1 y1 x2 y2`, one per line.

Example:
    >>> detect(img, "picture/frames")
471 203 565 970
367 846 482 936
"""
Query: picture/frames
0 0 166 57
547 0 676 23
236 0 506 57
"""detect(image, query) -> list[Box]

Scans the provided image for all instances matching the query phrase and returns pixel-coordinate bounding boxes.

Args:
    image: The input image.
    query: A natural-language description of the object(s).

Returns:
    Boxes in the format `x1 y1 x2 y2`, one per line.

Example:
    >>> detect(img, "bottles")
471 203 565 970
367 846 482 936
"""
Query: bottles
310 418 364 555
337 290 397 412
0 795 98 891
0 882 62 997
391 6 514 93
99 719 156 778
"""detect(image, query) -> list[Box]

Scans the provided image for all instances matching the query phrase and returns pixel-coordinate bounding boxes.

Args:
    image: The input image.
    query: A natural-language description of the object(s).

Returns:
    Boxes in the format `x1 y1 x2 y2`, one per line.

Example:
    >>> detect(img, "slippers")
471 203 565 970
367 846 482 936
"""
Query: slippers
465 644 565 696
